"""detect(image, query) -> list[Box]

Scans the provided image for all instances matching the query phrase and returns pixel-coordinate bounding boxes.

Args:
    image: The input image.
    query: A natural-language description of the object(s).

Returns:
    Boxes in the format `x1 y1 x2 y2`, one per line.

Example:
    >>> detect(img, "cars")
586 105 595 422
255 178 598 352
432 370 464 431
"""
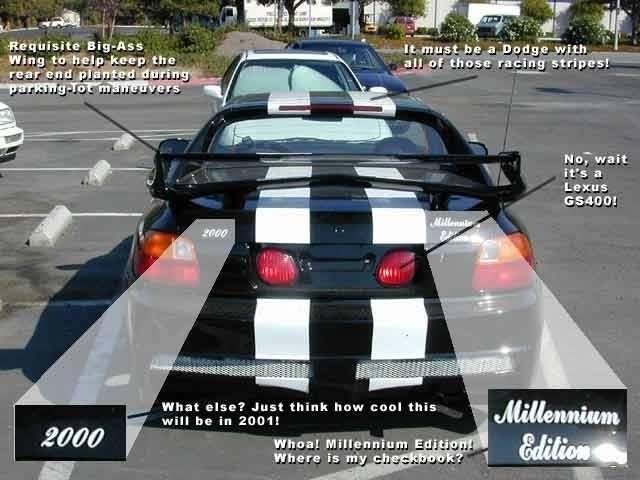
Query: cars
0 101 28 164
363 22 378 32
37 16 77 28
283 39 411 134
202 46 392 147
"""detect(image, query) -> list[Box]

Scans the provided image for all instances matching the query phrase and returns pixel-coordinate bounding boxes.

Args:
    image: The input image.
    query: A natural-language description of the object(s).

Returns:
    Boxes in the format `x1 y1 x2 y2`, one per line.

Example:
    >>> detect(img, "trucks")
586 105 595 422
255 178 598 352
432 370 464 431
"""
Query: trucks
219 0 334 38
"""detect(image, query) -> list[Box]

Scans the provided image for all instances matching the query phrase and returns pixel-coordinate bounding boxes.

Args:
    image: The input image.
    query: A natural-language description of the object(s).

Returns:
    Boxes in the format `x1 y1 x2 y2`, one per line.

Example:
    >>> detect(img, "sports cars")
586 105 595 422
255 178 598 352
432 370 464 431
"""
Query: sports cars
120 86 547 437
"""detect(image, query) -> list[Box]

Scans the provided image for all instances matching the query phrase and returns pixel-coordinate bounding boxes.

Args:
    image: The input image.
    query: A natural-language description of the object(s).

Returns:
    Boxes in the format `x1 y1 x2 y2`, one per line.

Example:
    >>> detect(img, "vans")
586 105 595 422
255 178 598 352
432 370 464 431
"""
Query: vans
385 16 415 38
474 13 523 42
